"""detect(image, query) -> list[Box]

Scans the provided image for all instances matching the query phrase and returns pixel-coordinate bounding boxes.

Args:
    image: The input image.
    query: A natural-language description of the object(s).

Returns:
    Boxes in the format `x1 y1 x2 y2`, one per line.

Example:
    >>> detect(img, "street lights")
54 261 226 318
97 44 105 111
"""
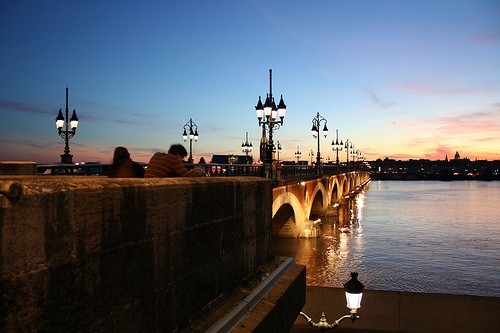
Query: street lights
255 68 287 178
55 87 80 163
331 130 366 164
295 145 331 163
274 141 284 160
241 132 253 157
183 118 199 163
310 111 328 162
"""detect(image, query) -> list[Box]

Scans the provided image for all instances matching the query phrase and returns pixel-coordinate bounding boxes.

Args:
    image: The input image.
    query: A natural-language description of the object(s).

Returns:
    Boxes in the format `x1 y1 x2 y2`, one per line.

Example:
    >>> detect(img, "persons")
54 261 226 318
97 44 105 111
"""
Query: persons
104 147 146 178
144 145 195 178
198 157 208 177
